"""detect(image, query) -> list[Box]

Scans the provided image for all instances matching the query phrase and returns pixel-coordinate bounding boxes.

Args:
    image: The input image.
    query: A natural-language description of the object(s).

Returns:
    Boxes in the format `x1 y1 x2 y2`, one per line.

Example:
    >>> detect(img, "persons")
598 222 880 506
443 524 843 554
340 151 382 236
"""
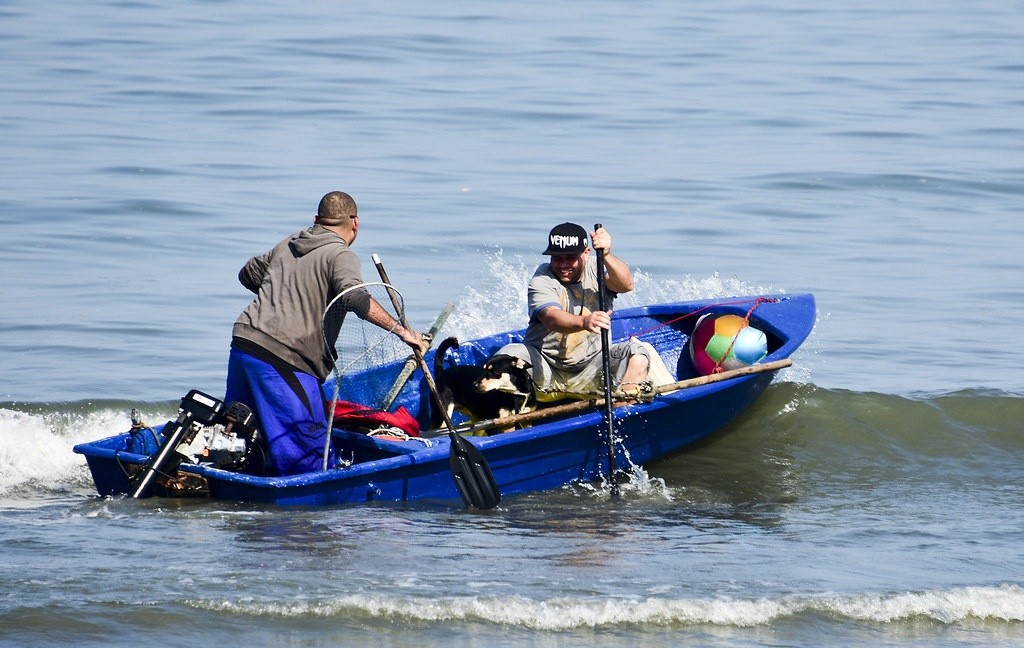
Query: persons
495 222 650 407
226 191 429 476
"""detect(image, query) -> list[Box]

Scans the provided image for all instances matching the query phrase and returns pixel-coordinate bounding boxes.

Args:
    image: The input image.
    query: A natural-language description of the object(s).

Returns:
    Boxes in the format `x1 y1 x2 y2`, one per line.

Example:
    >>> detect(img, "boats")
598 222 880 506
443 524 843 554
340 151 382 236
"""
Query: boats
71 293 819 512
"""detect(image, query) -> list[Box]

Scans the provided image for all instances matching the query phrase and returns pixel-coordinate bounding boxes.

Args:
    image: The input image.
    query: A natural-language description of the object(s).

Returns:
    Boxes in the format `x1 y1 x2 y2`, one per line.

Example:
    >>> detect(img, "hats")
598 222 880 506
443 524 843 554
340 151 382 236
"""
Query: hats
541 222 588 258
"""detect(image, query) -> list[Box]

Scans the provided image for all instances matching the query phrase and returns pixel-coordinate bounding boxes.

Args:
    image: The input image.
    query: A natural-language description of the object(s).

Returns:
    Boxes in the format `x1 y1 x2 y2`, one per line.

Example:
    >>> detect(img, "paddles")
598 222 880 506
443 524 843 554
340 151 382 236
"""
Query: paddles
594 222 632 497
371 253 502 511
415 358 794 439
